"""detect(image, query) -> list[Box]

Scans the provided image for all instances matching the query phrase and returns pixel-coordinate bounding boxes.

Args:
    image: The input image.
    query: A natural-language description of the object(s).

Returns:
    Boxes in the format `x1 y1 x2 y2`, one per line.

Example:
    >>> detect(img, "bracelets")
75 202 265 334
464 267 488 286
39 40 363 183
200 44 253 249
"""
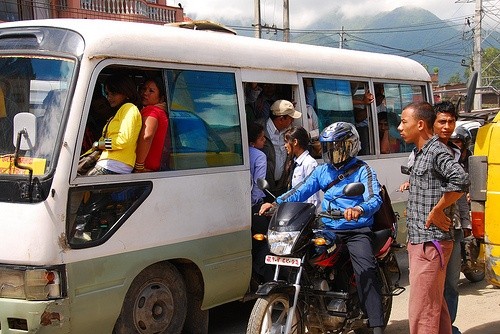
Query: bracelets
95 141 99 151
135 162 145 170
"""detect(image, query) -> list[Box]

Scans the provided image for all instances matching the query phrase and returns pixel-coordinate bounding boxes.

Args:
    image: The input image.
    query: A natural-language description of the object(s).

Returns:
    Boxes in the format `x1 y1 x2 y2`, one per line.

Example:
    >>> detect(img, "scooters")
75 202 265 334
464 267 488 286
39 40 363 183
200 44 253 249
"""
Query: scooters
461 211 488 283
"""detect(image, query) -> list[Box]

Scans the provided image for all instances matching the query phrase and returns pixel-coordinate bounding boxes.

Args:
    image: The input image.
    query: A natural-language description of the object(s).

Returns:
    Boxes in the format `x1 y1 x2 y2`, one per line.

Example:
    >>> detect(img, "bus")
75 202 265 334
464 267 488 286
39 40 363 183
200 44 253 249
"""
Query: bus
453 71 500 286
0 17 435 334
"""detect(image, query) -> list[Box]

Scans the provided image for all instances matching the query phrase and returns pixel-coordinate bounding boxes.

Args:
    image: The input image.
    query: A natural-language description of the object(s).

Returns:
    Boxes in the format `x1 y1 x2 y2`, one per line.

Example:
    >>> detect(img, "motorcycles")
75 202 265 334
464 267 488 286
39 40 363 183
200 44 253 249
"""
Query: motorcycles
247 178 406 332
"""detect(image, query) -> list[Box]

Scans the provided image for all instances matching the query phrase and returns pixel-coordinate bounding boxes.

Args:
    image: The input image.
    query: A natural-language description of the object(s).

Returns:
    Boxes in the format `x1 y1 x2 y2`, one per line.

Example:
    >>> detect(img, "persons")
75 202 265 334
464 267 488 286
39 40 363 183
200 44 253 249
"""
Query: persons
81 75 142 175
133 74 169 173
281 127 320 214
246 123 270 290
377 112 398 153
357 87 386 126
259 122 385 333
397 101 470 334
263 100 300 195
430 102 469 334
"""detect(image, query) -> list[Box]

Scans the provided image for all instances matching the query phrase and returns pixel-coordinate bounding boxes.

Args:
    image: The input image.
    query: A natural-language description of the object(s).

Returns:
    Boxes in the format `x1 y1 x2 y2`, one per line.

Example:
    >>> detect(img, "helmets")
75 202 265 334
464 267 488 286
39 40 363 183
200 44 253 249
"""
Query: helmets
450 126 472 142
319 121 362 164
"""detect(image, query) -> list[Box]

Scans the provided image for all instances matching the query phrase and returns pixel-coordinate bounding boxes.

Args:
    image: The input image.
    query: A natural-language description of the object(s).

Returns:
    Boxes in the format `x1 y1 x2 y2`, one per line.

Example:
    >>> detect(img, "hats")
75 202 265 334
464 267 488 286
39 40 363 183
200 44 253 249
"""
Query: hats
270 99 302 120
